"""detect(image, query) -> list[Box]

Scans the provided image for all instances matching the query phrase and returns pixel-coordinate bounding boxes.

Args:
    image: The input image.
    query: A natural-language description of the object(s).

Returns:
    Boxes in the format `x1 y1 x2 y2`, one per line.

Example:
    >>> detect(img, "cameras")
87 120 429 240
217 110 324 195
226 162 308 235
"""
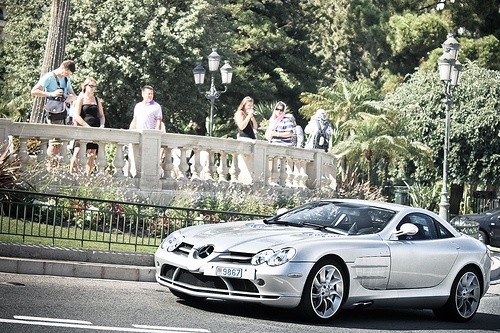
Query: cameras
54 88 66 101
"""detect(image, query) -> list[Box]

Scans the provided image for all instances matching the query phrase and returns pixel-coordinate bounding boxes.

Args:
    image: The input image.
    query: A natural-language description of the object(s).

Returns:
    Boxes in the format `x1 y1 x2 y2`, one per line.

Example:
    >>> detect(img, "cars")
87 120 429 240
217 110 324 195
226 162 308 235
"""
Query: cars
153 199 491 327
450 209 500 246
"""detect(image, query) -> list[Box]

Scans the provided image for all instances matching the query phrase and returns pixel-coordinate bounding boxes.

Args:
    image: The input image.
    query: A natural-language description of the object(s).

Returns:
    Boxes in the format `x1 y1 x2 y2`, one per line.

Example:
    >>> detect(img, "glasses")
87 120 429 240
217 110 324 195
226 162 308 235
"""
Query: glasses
276 108 284 112
87 84 95 88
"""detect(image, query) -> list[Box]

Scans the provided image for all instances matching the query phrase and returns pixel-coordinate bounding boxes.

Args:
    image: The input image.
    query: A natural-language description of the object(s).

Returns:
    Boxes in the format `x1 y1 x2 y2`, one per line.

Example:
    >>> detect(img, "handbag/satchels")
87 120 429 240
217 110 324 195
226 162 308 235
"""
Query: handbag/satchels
44 98 66 120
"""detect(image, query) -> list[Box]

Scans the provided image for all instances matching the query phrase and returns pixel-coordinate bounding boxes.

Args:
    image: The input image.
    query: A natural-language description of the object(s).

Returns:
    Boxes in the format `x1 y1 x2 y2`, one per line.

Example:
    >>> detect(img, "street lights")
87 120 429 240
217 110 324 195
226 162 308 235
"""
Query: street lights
192 45 233 136
438 31 464 235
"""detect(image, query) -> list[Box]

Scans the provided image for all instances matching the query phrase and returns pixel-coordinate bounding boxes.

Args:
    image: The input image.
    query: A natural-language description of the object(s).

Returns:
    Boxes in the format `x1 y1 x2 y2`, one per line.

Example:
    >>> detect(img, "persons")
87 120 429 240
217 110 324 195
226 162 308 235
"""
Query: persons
267 102 304 186
305 108 332 152
31 59 78 169
69 76 105 175
234 96 258 184
128 84 163 131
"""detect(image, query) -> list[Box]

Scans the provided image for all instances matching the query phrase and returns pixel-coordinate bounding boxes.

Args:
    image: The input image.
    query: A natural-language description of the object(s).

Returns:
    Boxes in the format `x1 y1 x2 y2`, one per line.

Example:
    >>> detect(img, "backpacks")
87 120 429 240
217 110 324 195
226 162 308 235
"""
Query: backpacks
313 121 330 152
